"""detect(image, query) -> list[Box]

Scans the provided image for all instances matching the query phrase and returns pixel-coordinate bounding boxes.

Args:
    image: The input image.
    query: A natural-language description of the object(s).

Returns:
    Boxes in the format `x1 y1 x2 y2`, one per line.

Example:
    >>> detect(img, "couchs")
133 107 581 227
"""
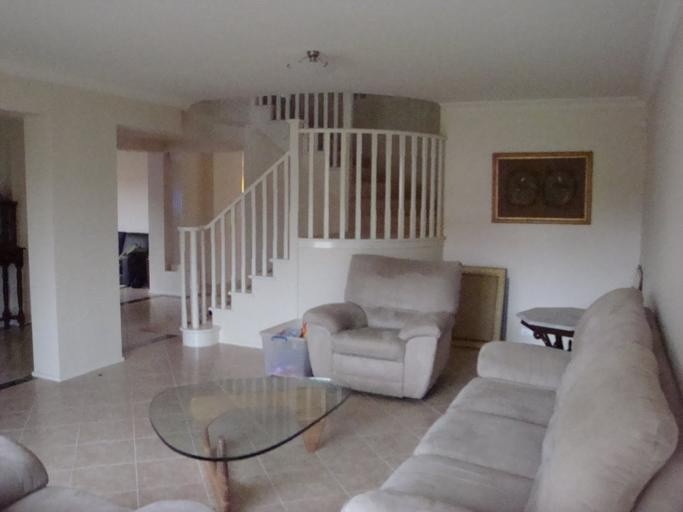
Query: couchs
340 284 682 512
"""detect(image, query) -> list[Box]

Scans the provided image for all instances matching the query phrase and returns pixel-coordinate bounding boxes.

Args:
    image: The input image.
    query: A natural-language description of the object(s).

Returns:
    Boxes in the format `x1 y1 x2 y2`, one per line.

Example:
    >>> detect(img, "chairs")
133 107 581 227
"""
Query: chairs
0 434 212 511
300 253 465 402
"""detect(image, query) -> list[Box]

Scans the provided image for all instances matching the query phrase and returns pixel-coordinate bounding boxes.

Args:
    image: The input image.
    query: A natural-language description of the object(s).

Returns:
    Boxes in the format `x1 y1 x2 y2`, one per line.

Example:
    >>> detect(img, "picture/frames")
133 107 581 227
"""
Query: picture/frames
448 265 506 350
489 151 592 224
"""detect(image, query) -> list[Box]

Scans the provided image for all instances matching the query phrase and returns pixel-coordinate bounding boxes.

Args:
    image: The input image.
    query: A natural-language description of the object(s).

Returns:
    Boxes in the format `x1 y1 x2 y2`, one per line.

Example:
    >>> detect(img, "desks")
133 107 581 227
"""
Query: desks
516 307 587 349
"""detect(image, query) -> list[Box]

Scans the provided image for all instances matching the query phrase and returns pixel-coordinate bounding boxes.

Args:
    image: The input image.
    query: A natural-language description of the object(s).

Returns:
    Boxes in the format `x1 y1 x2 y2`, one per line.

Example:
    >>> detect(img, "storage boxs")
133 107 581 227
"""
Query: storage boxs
258 315 310 380
